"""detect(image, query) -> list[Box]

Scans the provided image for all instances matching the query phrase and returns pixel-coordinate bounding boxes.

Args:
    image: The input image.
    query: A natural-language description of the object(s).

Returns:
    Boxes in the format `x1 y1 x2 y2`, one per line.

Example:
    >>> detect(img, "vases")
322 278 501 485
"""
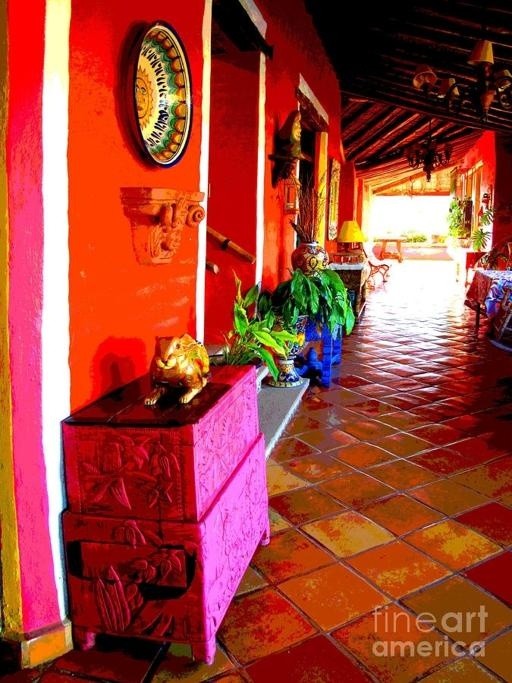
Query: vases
266 313 308 387
289 240 329 276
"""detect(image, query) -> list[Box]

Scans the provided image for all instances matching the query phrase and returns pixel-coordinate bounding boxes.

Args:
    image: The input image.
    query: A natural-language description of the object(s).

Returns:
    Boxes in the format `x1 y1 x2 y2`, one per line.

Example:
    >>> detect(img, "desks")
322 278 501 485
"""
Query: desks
372 237 408 262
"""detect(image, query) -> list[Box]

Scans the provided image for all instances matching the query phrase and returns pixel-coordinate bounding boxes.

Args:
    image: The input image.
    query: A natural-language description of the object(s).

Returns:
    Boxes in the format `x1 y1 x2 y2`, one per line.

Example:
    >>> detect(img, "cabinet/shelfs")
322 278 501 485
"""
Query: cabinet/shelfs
60 366 271 664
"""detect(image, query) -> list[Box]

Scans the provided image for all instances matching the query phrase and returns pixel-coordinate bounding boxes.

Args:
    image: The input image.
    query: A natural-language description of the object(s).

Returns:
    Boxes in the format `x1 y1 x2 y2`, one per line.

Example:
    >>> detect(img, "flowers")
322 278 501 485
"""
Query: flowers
470 210 496 249
446 199 463 236
283 168 338 243
211 276 304 384
268 272 357 336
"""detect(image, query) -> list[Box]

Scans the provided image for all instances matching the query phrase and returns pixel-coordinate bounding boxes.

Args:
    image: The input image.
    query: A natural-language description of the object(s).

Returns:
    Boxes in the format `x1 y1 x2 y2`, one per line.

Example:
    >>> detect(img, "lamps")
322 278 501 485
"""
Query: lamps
406 117 452 182
337 220 367 252
411 17 512 125
